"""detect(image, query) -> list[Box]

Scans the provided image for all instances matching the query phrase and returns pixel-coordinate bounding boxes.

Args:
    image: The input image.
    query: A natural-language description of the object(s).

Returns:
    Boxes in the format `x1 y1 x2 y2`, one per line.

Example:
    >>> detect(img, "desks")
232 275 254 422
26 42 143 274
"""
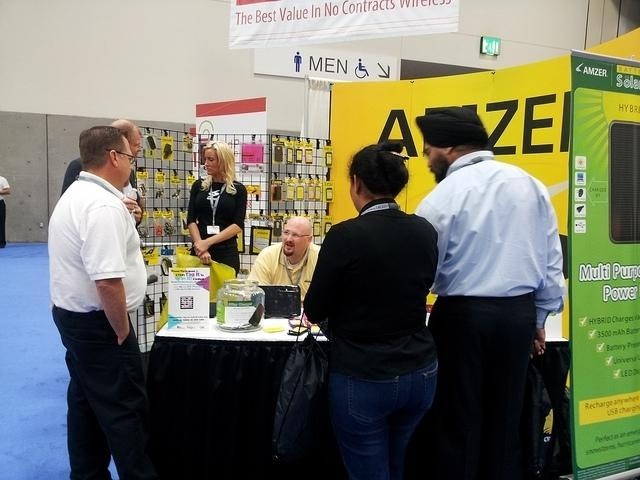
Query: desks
151 314 570 479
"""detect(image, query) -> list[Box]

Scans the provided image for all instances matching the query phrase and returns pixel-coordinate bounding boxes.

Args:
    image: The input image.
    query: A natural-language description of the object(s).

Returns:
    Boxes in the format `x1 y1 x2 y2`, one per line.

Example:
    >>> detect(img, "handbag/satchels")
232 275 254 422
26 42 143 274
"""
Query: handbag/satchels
270 335 326 461
523 363 554 477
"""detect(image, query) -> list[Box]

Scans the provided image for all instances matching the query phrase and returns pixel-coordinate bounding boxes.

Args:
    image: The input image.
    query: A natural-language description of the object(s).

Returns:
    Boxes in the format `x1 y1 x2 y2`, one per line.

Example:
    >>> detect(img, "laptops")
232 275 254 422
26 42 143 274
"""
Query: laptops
260 285 302 319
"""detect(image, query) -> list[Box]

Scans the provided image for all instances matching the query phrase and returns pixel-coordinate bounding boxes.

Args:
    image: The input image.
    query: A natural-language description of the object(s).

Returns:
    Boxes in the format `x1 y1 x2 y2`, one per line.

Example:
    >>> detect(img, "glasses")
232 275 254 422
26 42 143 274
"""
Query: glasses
106 148 136 165
282 229 310 238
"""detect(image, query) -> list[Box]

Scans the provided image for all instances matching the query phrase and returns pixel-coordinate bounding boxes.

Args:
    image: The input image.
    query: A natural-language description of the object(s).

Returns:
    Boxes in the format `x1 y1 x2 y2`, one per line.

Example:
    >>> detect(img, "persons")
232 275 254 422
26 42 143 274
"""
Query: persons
46 126 161 480
248 216 322 308
60 118 145 230
0 175 11 249
302 139 439 480
185 139 248 277
413 106 567 480
